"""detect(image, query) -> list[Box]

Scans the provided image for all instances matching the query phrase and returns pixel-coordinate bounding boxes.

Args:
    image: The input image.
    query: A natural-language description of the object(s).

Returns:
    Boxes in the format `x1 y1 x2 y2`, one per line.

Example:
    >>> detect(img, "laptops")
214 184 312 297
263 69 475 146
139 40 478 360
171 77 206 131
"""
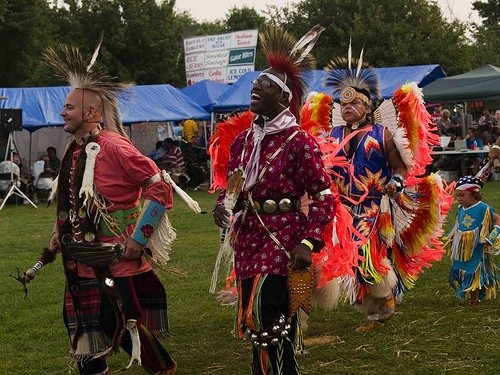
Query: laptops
454 140 468 151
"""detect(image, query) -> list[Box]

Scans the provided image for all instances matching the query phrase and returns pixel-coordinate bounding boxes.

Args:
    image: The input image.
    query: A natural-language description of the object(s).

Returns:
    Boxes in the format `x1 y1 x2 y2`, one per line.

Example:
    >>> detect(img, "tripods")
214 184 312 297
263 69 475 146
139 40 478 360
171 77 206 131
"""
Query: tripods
0 133 37 210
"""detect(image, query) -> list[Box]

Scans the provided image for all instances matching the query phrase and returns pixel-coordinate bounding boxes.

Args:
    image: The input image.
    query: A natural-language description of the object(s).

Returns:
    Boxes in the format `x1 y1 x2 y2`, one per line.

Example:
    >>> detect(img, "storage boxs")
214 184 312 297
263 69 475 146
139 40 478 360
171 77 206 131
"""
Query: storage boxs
440 171 459 183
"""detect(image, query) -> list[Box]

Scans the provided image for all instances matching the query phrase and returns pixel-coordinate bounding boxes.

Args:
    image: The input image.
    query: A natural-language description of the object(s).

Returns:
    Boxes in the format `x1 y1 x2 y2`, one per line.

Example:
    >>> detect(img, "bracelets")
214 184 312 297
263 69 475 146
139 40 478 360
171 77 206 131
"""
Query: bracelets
492 144 500 151
301 239 313 250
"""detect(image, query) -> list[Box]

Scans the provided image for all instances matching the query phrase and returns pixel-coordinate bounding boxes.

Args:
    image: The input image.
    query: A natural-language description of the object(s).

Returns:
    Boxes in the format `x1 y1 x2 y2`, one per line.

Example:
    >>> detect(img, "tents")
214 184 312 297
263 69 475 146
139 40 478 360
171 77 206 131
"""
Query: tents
179 64 447 145
0 84 211 169
422 64 500 137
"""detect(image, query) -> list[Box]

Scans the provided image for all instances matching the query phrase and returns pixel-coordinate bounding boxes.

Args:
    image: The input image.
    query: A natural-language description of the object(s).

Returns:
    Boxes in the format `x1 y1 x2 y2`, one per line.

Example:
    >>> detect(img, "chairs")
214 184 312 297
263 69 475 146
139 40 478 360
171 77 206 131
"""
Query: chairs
0 173 20 207
35 171 56 204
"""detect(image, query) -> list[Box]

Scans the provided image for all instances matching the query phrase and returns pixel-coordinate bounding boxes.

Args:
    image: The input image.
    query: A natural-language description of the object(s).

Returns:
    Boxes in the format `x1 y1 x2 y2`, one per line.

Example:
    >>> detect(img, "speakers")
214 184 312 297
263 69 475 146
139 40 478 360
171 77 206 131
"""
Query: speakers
1 108 23 132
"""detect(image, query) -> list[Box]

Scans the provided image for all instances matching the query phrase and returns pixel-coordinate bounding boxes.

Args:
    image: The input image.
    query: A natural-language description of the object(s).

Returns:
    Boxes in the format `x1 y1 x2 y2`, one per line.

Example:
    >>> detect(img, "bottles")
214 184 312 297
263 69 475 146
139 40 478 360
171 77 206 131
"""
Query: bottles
21 170 25 178
471 140 477 149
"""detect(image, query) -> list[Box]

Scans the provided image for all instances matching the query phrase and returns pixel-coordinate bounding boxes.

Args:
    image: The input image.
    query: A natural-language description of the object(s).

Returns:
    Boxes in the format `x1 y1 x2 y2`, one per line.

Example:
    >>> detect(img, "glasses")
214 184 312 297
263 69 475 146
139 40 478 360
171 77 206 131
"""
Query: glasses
251 79 277 88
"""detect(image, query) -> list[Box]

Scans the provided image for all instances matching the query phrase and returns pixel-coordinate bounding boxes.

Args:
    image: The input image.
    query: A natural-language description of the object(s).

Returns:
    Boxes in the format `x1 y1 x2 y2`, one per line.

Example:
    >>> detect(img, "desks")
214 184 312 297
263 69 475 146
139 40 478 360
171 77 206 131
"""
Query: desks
431 148 489 176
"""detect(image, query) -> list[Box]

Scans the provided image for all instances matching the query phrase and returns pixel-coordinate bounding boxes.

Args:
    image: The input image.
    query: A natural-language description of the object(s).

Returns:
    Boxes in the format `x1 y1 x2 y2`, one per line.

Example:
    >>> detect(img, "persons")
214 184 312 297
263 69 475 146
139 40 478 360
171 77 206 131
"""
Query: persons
443 175 500 309
149 136 205 191
32 147 61 203
326 85 407 322
8 152 20 164
429 106 500 165
48 88 178 375
173 119 198 141
212 66 336 375
